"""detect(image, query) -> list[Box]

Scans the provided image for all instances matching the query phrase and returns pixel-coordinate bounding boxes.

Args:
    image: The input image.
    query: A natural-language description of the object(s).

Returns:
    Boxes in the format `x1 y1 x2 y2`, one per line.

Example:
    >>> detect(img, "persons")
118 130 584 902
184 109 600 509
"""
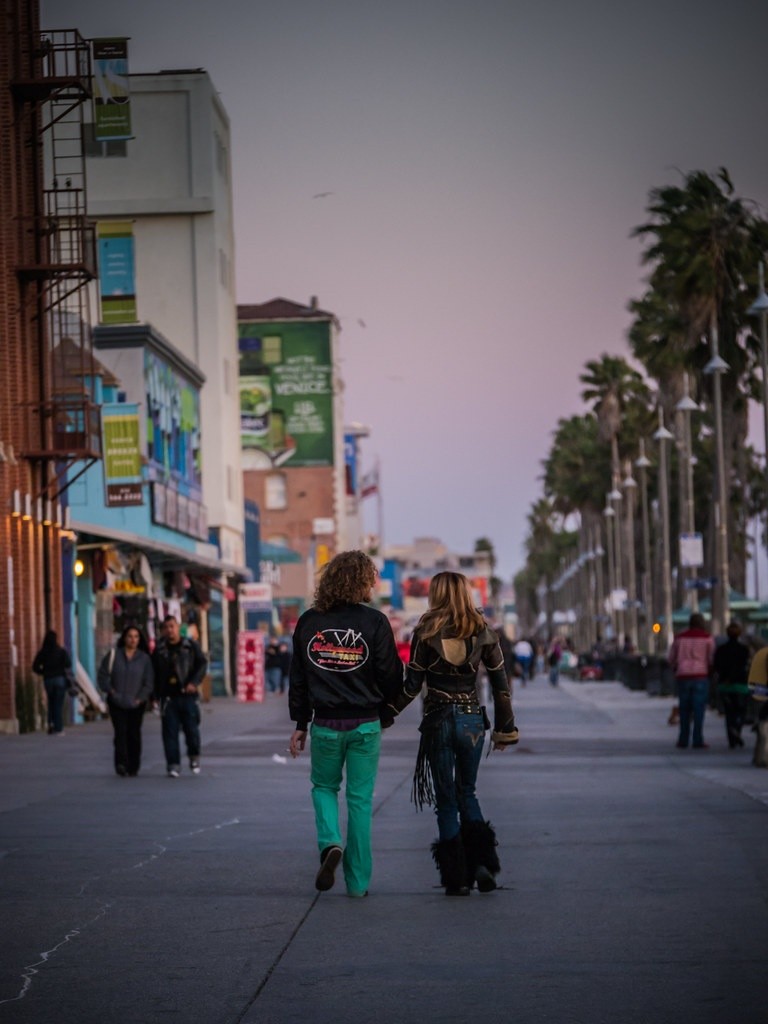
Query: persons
591 636 618 652
386 571 519 896
491 622 512 684
565 638 574 651
288 550 404 896
150 616 210 778
711 623 751 748
548 637 563 685
623 637 633 653
747 647 768 765
513 635 537 688
97 625 153 777
264 636 292 693
32 631 71 735
667 612 717 750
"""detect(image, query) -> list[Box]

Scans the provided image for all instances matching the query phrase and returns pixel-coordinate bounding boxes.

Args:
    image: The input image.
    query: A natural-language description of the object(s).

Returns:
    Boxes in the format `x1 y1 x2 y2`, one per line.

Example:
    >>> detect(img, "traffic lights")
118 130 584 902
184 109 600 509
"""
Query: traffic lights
652 623 660 634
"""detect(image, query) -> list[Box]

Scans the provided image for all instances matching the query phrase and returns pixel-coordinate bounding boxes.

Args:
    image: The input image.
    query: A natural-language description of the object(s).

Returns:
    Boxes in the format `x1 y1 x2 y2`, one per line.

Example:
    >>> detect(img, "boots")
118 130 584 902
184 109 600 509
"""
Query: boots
432 830 475 895
463 820 499 892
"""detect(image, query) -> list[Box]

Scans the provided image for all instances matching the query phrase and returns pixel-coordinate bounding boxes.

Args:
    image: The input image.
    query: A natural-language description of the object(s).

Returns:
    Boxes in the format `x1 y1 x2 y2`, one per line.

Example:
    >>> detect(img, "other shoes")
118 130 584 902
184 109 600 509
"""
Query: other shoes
169 770 179 777
677 743 687 748
694 744 709 749
192 760 200 774
315 845 342 891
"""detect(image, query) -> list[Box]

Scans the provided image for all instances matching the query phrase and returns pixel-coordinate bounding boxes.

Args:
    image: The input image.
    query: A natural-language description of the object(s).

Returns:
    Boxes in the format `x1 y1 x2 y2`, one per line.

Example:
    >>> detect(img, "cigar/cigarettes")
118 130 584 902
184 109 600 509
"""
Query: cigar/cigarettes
285 749 304 752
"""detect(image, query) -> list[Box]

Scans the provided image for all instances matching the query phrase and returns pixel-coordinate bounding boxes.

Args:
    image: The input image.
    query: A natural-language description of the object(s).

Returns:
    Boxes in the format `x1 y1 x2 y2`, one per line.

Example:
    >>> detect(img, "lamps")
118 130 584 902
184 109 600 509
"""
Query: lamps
241 439 297 468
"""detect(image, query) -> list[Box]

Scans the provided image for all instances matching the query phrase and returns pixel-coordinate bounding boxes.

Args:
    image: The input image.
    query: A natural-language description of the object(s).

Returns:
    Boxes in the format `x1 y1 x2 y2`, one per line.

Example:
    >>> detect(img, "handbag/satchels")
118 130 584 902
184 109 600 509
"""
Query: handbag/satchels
68 685 79 696
